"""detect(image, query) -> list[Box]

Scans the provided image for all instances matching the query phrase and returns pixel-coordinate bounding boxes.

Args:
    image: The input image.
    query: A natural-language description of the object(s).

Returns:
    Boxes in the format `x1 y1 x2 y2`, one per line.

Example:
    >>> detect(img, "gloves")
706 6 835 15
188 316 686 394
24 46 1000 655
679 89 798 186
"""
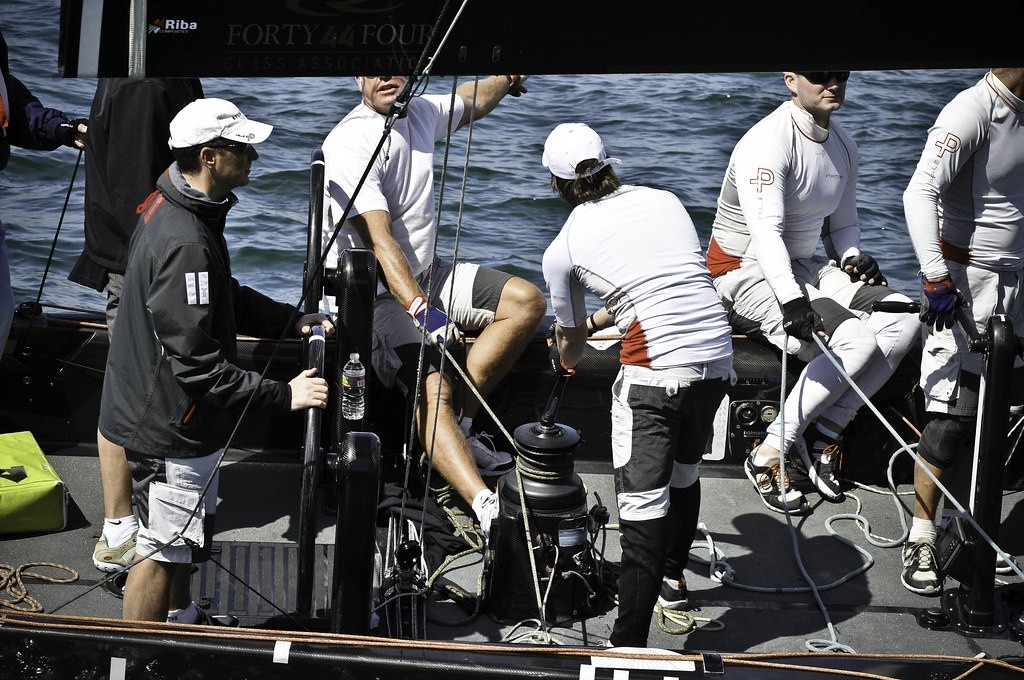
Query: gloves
406 295 463 351
919 277 972 331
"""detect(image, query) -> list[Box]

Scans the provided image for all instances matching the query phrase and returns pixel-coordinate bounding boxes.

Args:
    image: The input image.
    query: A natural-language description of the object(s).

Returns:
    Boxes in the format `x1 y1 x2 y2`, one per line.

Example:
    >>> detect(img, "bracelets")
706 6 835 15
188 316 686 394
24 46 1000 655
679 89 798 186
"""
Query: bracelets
589 312 599 330
505 74 514 93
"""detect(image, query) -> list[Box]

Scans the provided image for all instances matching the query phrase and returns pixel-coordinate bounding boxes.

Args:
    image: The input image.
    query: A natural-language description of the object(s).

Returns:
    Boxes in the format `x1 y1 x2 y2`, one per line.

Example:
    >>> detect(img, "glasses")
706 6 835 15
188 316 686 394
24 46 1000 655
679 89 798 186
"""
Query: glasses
793 71 852 84
200 140 253 155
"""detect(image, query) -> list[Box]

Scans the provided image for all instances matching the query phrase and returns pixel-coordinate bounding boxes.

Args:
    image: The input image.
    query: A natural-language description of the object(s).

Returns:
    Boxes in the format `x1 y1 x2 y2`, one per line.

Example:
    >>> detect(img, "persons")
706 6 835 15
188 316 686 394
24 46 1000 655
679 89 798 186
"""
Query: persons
0 31 91 361
902 67 1024 596
544 122 734 648
319 76 544 539
707 72 922 515
93 98 336 635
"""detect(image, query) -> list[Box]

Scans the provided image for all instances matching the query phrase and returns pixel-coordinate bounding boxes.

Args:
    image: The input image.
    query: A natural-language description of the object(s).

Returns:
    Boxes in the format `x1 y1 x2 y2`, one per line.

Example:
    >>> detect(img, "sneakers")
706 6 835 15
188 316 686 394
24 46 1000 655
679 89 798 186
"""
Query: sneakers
743 442 811 514
899 537 942 595
92 525 139 575
797 421 846 502
164 606 240 632
466 431 517 477
655 576 688 610
994 550 1017 575
480 490 502 540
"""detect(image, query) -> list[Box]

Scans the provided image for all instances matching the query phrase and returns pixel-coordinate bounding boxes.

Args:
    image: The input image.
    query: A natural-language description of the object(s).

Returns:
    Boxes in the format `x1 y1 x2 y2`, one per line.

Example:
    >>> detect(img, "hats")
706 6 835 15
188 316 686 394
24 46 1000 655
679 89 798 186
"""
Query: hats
540 121 623 180
167 97 274 151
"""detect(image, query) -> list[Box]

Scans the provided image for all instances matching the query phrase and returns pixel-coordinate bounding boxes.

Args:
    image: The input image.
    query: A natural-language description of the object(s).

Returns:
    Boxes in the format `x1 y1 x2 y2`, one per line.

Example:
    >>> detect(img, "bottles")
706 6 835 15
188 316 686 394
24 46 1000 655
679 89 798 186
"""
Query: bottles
342 353 364 420
